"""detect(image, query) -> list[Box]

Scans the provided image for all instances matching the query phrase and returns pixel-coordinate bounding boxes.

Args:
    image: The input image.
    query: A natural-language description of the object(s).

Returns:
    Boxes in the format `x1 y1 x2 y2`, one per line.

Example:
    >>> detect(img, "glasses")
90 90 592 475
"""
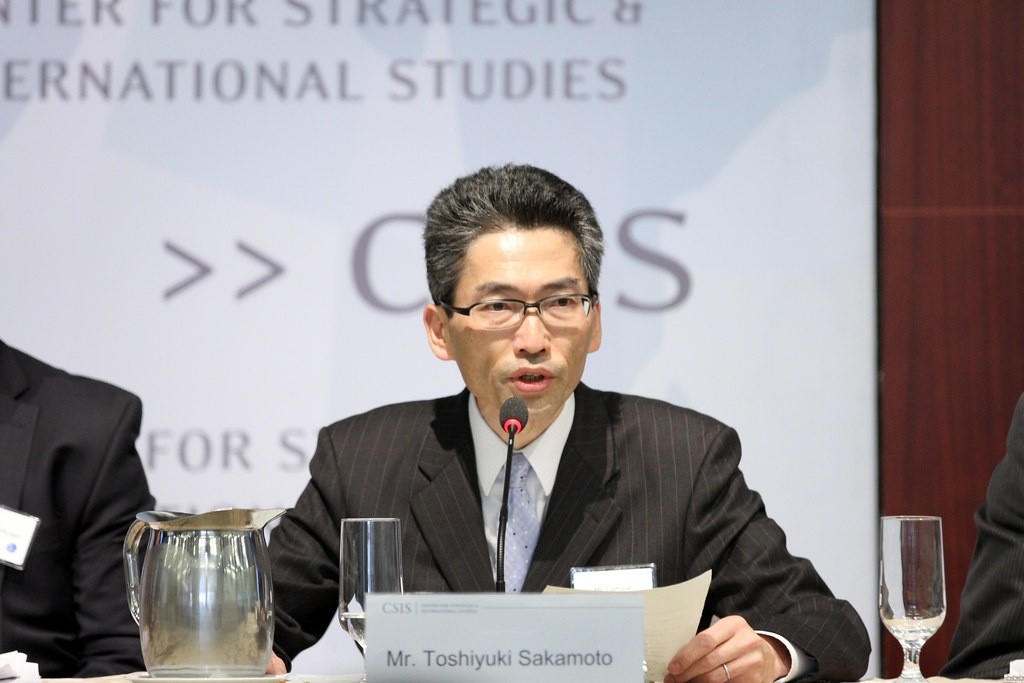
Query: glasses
438 294 596 329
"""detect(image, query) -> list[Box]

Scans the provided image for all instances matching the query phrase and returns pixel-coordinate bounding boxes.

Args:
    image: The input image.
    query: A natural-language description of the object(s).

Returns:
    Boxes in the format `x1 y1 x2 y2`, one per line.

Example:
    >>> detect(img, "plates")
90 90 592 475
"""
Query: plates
126 670 289 683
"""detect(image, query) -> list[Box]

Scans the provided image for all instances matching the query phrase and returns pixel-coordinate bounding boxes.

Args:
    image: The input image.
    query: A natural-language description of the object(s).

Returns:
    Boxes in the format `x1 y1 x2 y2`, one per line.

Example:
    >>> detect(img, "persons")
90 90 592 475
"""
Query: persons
1 332 164 678
255 159 873 683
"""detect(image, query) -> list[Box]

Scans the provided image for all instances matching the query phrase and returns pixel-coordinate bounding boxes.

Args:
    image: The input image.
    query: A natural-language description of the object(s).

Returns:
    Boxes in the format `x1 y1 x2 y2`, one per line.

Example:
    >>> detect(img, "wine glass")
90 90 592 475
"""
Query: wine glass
878 512 947 682
340 514 404 683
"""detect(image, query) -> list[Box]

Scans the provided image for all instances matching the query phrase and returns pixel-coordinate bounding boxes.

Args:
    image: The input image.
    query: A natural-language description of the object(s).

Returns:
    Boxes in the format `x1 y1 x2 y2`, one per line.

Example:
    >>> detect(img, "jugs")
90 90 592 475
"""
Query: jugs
124 502 302 675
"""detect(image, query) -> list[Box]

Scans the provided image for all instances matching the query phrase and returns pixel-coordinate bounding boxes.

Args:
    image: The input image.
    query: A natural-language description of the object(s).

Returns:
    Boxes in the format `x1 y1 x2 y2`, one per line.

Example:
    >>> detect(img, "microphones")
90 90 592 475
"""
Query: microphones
493 397 530 591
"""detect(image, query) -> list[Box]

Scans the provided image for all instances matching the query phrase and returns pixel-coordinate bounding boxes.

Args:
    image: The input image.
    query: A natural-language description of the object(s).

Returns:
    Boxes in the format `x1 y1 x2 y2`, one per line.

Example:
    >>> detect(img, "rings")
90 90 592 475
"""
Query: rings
721 663 733 681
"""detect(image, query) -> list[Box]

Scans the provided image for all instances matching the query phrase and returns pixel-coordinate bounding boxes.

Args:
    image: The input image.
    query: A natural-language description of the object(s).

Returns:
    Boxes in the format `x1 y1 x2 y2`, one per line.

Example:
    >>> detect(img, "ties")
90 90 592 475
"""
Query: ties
492 453 540 594
